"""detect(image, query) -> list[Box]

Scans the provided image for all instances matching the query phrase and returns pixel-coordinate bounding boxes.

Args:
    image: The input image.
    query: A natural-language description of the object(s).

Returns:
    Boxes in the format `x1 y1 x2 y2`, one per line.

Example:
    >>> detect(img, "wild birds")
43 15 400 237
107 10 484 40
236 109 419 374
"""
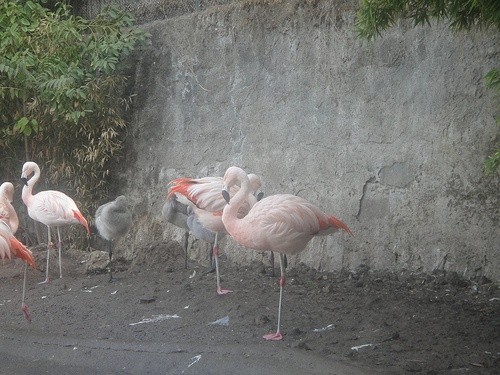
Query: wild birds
94 194 135 283
20 161 91 285
0 207 37 322
221 166 356 341
162 173 264 295
0 182 19 235
